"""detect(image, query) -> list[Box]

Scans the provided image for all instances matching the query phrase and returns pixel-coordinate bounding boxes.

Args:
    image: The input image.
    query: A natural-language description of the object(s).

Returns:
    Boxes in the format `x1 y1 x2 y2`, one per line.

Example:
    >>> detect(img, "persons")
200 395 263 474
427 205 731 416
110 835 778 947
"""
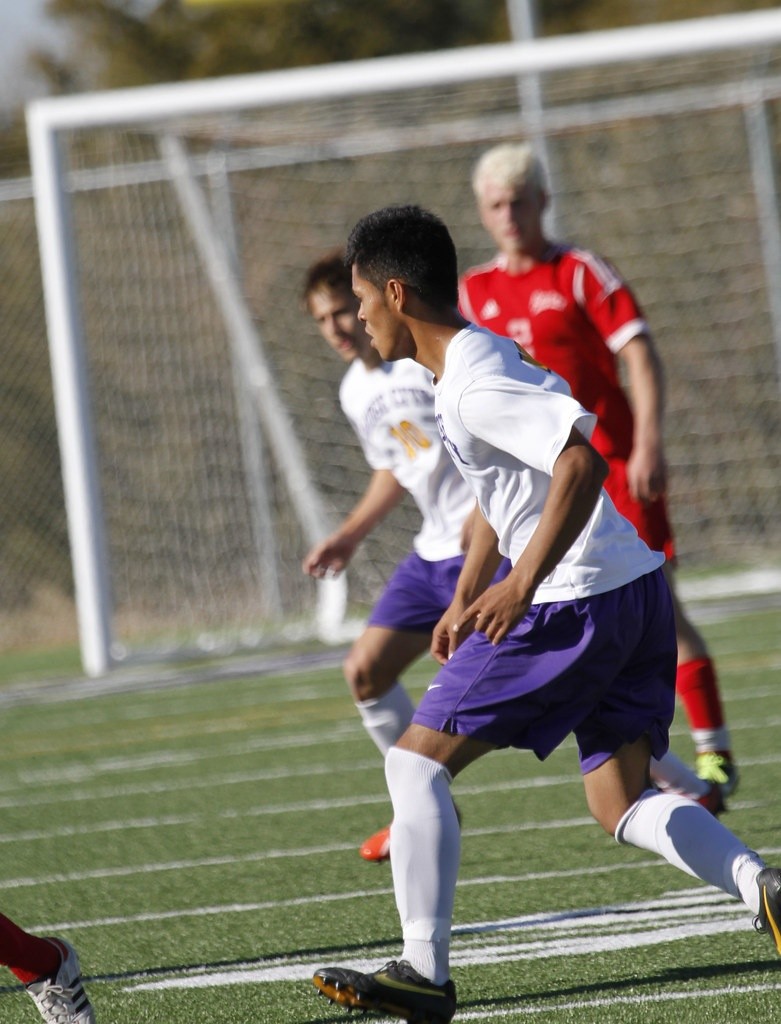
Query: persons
300 254 728 866
457 144 742 797
313 204 781 1024
0 912 96 1024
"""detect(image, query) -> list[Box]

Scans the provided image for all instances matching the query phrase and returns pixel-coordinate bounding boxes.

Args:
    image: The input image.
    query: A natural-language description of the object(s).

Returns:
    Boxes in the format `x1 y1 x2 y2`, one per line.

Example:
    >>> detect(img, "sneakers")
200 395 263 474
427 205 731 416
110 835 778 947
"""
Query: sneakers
312 958 457 1024
693 750 742 797
680 778 728 819
755 866 781 960
359 794 463 866
24 936 94 1024
